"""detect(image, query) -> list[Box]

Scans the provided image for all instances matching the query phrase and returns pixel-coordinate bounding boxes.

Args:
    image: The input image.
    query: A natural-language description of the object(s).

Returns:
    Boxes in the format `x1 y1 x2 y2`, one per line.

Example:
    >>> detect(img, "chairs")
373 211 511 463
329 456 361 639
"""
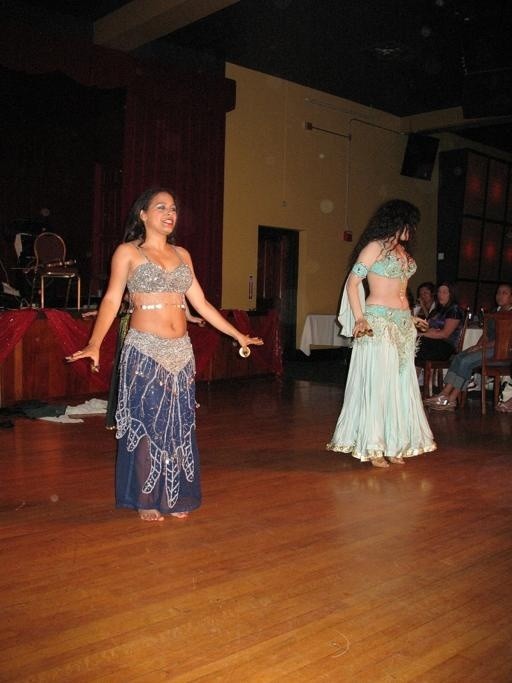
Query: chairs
480 311 512 415
412 312 471 407
26 230 80 307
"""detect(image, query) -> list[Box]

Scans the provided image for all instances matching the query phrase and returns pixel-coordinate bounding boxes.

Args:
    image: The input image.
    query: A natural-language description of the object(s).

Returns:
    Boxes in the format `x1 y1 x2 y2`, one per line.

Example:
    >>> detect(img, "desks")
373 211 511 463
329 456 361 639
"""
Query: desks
298 312 354 368
461 318 486 356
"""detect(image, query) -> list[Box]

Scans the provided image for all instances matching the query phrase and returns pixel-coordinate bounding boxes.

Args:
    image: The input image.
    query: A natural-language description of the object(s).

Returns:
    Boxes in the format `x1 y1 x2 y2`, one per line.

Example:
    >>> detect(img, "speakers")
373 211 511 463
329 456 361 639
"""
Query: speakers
400 133 440 181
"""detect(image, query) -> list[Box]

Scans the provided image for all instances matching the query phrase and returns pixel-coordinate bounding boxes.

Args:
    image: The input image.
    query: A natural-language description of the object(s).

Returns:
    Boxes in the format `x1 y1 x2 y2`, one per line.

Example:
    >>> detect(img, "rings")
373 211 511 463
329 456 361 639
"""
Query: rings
77 349 84 354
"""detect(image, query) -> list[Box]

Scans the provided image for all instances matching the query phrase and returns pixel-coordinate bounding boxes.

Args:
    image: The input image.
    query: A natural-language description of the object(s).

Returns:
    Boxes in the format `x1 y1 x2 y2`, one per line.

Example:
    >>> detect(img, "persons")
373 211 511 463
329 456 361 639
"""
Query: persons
419 281 512 412
59 186 265 526
321 196 439 471
414 280 464 379
412 280 437 322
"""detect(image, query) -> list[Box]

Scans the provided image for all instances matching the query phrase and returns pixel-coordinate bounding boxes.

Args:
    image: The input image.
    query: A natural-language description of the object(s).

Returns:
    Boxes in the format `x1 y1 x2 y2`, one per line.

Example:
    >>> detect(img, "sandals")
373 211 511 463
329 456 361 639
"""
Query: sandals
422 394 458 411
